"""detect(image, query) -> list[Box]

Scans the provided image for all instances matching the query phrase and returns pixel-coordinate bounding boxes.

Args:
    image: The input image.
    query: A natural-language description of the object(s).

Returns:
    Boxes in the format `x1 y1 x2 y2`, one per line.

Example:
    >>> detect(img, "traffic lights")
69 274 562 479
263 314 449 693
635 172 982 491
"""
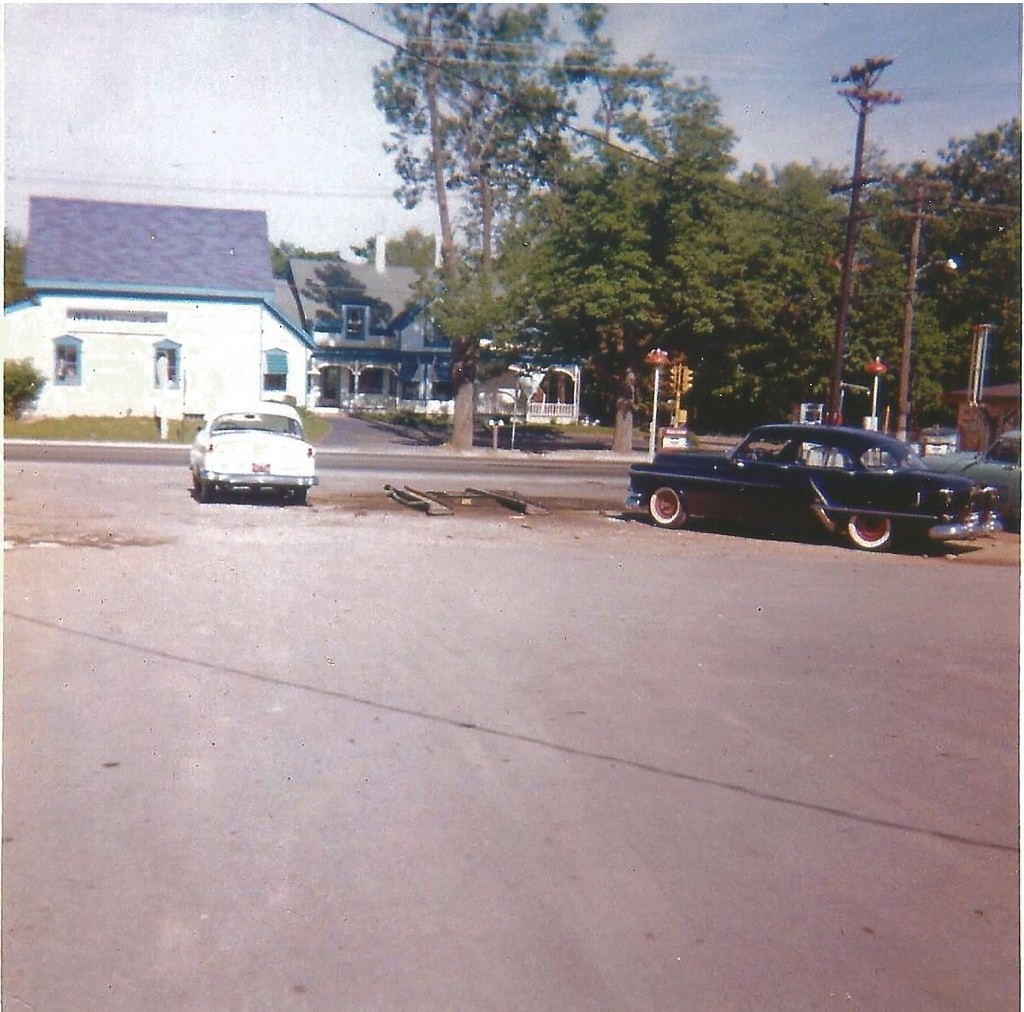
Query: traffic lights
669 365 679 393
681 366 695 393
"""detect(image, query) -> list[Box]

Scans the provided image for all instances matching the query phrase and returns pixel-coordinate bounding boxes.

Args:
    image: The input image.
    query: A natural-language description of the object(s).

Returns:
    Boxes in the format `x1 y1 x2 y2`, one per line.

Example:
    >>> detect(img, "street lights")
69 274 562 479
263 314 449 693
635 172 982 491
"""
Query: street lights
863 355 887 430
892 257 958 445
643 347 672 463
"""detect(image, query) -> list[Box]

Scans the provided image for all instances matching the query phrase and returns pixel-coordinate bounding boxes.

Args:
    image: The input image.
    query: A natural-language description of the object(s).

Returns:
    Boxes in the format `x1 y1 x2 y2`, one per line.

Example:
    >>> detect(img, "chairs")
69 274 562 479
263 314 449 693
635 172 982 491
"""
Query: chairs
775 442 803 464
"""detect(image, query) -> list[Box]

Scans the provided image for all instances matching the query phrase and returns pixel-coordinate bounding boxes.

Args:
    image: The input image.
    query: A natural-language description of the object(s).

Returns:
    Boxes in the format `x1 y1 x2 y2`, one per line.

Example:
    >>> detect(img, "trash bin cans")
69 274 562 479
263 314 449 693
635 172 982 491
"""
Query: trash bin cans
657 426 688 453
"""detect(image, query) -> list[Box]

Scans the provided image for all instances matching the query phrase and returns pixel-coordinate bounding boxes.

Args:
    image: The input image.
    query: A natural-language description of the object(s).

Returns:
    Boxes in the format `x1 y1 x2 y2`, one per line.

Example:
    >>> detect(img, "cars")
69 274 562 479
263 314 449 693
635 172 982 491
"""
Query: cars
884 428 1020 522
188 400 320 501
623 421 1004 554
